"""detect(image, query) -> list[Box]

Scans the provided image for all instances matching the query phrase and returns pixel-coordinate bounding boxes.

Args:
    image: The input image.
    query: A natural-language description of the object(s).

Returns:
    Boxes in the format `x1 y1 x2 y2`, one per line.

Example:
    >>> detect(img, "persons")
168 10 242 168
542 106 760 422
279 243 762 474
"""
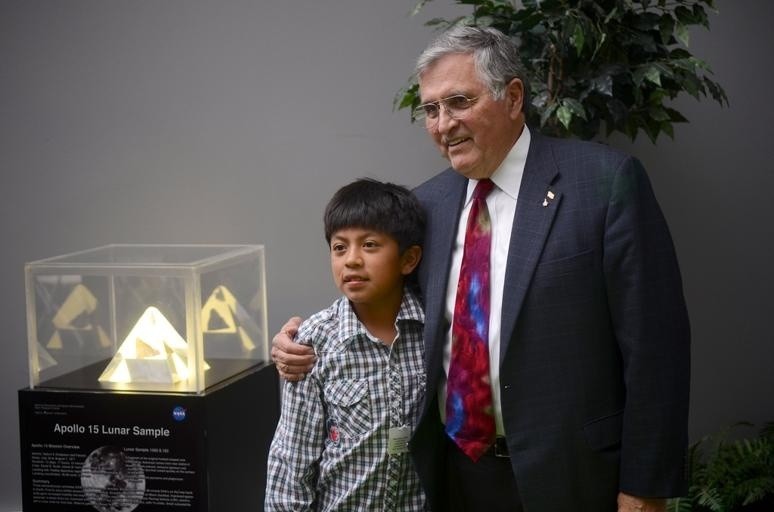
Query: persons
269 18 692 511
262 175 432 512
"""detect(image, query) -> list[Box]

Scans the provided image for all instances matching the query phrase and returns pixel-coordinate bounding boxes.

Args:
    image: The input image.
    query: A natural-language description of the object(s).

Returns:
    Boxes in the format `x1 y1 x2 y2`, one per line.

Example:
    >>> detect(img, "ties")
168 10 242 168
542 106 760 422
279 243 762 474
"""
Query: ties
443 178 498 466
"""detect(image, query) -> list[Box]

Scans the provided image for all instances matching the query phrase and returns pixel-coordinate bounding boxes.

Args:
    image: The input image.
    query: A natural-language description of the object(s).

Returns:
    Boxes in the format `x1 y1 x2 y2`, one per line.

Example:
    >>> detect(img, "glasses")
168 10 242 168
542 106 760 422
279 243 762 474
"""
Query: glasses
413 85 493 129
